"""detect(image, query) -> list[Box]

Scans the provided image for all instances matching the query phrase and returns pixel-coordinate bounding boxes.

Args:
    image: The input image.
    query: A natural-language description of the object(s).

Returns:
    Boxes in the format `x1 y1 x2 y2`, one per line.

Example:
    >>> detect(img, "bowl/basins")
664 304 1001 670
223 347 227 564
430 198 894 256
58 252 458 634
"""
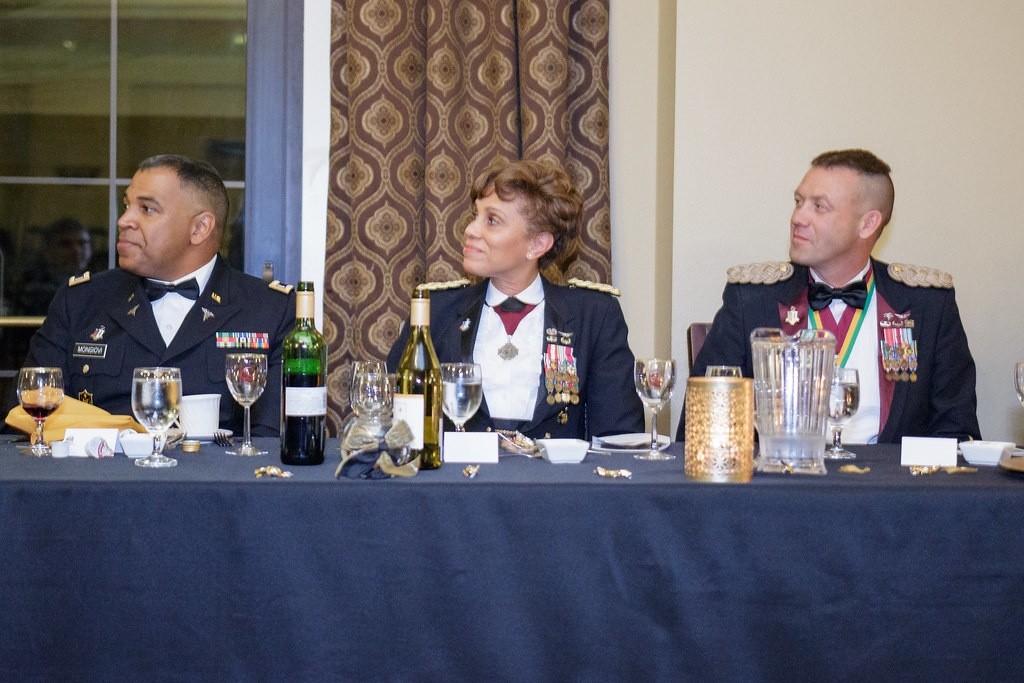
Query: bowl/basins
121 435 153 457
536 438 589 465
960 441 1016 465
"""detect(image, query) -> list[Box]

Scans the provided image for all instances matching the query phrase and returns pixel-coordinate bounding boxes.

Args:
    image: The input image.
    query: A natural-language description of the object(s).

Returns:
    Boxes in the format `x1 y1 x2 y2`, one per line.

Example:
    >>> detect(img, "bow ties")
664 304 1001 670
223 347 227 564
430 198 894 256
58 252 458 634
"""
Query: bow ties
808 282 868 311
143 279 199 301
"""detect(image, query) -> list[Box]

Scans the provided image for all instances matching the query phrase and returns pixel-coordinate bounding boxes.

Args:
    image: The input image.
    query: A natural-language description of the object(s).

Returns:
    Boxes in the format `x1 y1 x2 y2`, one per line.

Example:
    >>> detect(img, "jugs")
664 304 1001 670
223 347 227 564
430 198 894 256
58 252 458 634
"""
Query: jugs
750 327 836 468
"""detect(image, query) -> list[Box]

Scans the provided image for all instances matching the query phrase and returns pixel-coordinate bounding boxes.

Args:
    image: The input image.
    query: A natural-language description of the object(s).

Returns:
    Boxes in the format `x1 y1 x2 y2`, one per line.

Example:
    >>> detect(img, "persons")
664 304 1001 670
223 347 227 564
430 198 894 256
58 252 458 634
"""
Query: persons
23 155 296 438
13 221 91 366
676 149 983 445
385 160 646 434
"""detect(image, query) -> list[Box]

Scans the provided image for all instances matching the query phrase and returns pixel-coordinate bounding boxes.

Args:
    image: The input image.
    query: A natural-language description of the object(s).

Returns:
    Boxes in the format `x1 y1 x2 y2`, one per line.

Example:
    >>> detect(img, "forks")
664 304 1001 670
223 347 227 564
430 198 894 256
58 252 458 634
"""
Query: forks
215 433 237 447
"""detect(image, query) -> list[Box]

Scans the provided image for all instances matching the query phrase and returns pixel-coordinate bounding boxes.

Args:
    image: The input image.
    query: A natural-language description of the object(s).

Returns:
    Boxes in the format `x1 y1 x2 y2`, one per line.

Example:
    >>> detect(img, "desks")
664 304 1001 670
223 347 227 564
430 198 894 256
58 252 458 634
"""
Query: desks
0 433 1024 683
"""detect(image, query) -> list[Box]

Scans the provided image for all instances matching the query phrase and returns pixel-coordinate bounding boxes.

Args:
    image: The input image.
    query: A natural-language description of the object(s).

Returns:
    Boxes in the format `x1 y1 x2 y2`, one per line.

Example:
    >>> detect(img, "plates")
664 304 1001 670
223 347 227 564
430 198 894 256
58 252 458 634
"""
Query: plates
182 428 233 440
592 434 669 451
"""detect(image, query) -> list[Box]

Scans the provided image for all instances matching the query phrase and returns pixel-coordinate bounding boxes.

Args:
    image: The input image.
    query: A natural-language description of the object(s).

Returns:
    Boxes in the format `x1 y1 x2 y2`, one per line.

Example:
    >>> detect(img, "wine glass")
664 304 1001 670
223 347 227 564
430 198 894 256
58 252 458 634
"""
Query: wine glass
1012 364 1023 457
17 369 65 456
825 368 860 459
132 367 182 467
634 359 678 460
225 354 268 456
440 364 483 432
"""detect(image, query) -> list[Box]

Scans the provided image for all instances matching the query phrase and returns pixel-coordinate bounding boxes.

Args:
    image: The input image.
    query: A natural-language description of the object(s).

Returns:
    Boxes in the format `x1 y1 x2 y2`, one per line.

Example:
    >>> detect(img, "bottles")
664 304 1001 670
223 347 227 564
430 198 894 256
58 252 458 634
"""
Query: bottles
394 288 440 469
278 279 327 467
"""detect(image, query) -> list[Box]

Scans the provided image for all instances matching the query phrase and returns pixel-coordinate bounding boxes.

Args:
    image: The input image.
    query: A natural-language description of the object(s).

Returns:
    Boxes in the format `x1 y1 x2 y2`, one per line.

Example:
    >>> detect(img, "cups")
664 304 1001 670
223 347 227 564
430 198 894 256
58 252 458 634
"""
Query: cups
174 395 221 435
704 365 742 380
347 358 394 421
